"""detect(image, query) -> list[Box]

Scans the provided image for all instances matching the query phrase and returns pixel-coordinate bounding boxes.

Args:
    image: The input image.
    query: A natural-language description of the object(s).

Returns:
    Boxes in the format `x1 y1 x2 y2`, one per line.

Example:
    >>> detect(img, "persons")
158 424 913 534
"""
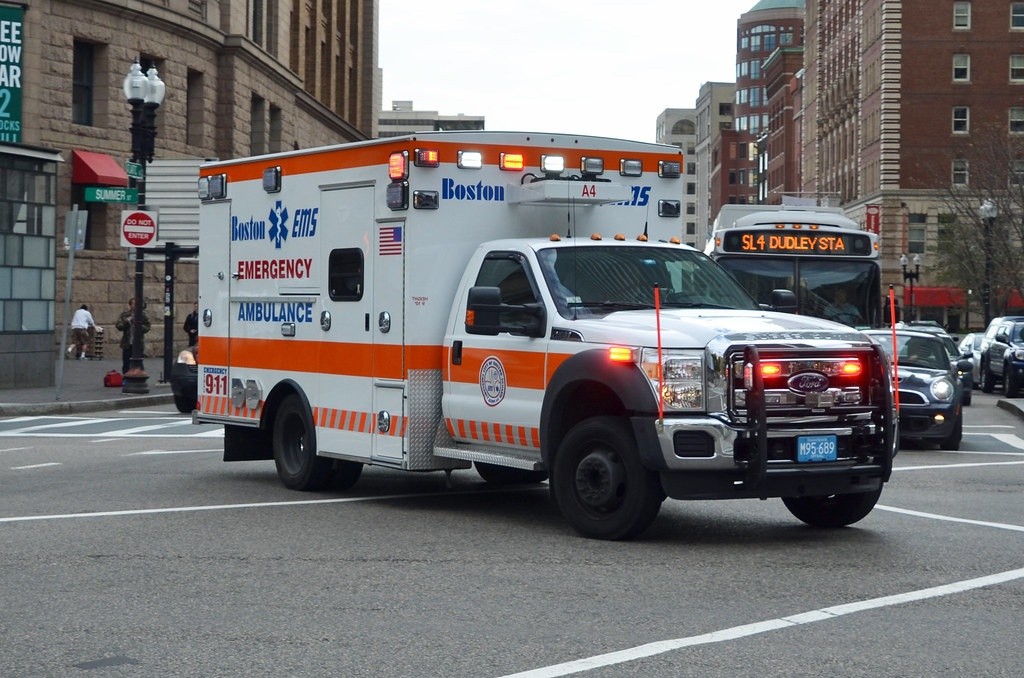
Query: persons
115 298 151 386
68 304 99 360
823 289 865 327
184 302 198 347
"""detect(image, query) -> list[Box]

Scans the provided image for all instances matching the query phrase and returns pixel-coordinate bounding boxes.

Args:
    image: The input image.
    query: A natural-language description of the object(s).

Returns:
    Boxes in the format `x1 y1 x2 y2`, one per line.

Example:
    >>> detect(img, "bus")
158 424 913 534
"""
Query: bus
703 203 883 332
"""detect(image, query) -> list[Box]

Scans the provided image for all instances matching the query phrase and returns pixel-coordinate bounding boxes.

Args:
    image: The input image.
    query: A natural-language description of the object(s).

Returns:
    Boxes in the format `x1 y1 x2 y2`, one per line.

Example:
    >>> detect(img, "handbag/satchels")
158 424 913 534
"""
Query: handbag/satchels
104 370 123 387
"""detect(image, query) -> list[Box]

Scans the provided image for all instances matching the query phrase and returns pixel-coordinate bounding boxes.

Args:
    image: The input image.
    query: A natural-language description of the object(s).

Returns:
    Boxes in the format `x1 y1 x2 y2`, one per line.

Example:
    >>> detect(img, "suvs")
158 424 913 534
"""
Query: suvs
978 316 1024 397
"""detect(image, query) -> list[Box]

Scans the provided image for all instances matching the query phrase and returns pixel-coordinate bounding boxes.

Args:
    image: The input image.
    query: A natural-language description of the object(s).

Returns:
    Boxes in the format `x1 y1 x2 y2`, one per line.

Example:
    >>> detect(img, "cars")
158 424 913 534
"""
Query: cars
171 341 198 414
858 329 973 451
891 320 974 406
959 333 985 390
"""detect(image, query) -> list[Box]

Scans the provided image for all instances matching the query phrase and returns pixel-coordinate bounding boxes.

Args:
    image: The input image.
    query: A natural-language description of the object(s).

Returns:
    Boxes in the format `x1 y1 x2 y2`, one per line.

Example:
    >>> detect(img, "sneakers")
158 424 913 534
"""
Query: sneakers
78 357 88 361
65 350 76 359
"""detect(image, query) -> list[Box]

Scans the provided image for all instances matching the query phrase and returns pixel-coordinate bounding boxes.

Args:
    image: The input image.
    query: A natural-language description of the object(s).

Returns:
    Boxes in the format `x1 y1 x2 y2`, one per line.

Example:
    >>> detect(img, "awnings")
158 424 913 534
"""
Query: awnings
71 151 128 187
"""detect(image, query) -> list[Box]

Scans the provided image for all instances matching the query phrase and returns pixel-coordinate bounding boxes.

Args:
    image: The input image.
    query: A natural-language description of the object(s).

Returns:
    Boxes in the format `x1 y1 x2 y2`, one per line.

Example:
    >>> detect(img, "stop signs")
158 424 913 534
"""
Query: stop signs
119 209 158 249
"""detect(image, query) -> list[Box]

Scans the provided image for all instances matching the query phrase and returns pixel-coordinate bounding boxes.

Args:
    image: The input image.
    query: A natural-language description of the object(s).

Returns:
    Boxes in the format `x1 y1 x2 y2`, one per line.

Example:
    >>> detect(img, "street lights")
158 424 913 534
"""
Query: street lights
979 197 998 332
899 252 921 321
120 54 166 393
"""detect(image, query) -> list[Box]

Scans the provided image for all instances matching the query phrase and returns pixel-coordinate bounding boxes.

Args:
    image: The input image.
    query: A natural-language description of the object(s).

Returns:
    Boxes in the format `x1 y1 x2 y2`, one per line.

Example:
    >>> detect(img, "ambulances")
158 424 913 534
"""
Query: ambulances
192 129 900 542
